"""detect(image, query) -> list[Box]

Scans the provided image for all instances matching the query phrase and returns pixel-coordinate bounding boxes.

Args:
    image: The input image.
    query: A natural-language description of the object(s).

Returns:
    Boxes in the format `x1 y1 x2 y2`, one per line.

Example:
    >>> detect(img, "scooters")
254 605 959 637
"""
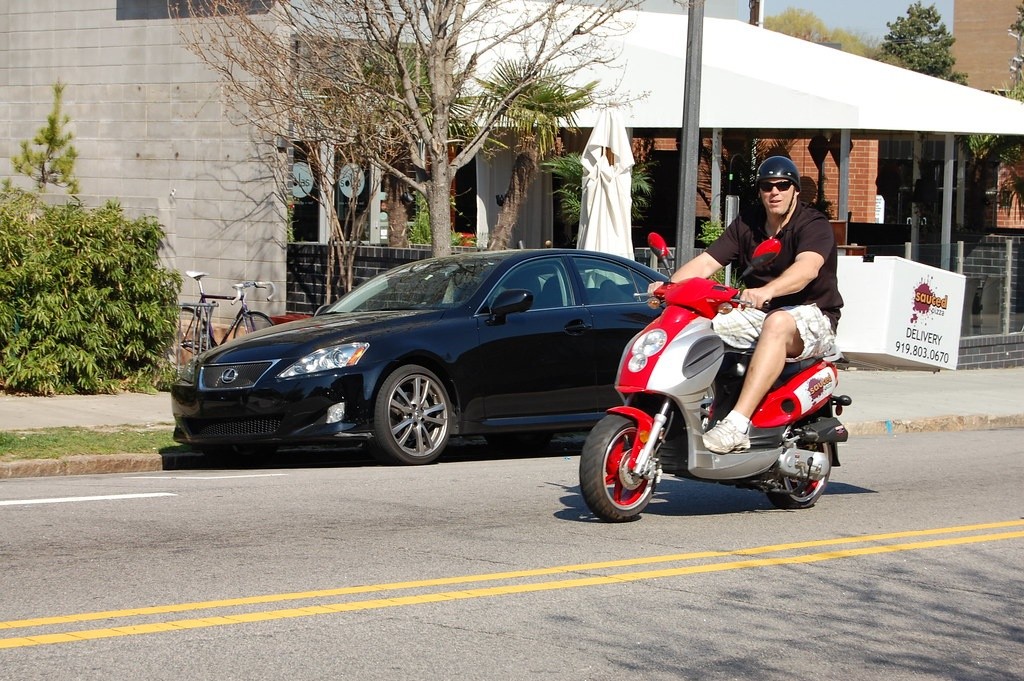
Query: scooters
580 231 851 527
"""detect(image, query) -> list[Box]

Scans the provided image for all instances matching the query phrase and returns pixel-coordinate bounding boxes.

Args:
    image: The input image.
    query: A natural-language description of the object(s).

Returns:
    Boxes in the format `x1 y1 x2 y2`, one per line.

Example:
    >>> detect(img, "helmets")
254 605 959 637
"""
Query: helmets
755 156 801 192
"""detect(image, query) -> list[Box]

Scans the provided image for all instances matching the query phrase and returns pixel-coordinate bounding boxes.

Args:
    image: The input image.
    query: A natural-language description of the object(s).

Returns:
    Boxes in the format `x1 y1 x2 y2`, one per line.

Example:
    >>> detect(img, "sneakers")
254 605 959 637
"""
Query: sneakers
702 420 751 455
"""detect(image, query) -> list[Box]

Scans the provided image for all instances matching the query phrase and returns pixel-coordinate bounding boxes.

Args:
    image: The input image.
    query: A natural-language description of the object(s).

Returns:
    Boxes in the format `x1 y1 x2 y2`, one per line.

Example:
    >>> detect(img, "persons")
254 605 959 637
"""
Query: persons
645 157 845 454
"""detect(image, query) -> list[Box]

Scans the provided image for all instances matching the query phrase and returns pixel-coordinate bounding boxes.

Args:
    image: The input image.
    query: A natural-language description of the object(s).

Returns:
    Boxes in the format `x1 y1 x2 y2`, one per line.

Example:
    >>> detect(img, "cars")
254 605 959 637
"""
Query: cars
171 247 671 461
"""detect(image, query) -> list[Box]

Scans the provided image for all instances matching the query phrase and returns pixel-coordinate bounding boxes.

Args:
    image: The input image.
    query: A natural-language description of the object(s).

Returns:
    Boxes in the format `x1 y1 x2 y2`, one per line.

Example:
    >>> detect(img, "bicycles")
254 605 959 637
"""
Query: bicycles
174 270 276 386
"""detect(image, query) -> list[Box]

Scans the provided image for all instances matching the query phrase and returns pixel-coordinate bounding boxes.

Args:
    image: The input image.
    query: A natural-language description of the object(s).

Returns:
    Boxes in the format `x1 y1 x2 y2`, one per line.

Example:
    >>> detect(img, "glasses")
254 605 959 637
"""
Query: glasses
758 181 793 192
